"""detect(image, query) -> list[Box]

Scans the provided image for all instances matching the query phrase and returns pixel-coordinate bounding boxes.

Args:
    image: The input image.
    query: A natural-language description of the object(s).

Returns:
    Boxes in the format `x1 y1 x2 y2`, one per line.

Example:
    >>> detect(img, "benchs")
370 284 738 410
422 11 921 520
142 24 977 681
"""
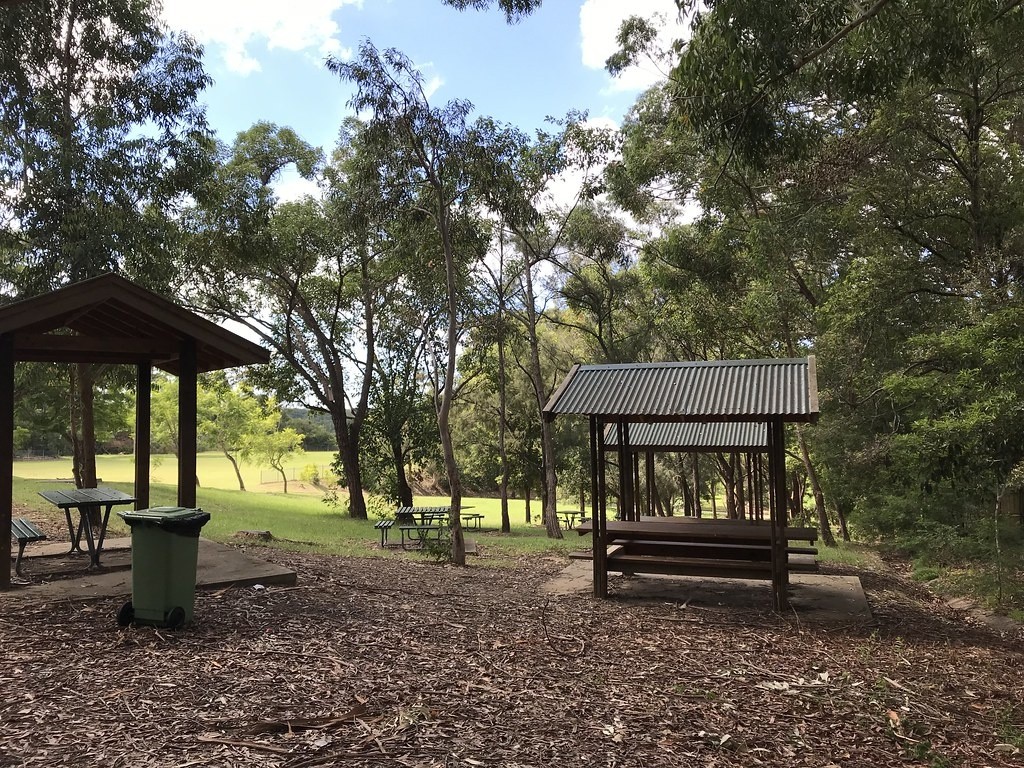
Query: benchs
10 518 47 577
569 538 819 580
374 514 484 548
559 520 569 530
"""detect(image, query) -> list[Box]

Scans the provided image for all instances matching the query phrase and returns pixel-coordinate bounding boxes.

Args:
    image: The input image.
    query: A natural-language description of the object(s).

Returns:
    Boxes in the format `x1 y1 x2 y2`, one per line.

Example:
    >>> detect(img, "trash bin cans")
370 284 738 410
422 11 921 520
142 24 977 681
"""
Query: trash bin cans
117 507 212 631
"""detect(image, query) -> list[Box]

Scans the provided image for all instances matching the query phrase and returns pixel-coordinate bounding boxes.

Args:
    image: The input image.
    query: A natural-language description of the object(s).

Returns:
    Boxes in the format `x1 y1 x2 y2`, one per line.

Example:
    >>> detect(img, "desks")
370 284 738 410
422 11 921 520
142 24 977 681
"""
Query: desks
38 487 141 569
556 511 585 529
396 507 448 544
576 520 818 551
